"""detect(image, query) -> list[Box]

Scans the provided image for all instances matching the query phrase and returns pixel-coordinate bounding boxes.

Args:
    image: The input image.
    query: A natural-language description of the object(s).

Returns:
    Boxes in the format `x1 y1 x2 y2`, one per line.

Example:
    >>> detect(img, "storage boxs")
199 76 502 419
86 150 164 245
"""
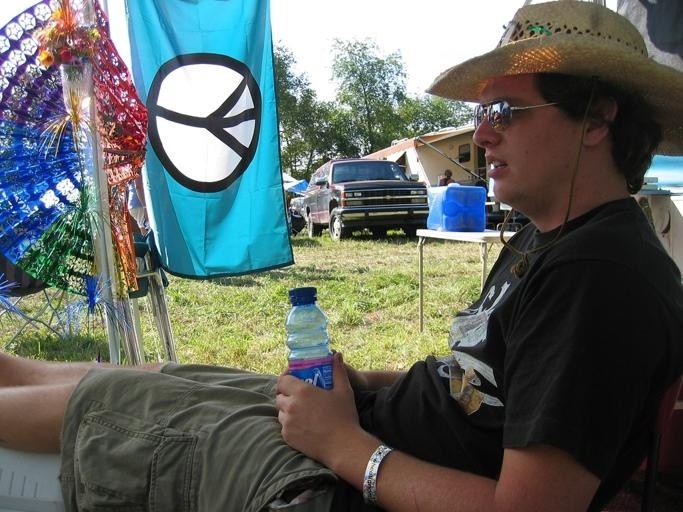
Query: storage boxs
426 185 487 232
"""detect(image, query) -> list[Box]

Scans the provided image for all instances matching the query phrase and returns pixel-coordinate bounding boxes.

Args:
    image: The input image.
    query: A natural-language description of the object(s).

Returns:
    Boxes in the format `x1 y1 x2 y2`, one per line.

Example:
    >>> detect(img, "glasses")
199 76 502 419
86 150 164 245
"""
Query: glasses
473 100 561 132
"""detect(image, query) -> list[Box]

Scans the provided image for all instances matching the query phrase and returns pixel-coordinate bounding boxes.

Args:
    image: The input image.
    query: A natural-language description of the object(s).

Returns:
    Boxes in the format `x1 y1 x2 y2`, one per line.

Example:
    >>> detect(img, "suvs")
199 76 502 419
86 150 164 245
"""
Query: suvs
298 155 430 243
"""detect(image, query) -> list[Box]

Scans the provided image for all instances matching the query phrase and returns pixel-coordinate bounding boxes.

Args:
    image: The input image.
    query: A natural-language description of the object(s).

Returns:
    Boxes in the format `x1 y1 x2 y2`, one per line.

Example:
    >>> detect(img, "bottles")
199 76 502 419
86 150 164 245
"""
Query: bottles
284 287 335 392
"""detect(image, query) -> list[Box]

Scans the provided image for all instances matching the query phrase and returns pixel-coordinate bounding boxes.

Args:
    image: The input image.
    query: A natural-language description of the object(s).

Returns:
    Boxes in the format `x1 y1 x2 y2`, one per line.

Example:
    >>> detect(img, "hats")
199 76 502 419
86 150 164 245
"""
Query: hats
424 0 682 156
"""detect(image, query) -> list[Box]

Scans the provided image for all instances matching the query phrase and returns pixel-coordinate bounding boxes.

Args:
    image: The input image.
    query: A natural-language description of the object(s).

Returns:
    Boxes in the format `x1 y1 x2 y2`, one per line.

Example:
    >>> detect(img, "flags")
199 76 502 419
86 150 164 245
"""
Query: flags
122 0 293 281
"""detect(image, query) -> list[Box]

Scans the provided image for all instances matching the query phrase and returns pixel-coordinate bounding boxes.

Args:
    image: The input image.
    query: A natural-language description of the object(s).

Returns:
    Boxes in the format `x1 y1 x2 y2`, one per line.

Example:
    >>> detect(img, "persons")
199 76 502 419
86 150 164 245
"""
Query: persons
0 1 682 512
437 169 456 187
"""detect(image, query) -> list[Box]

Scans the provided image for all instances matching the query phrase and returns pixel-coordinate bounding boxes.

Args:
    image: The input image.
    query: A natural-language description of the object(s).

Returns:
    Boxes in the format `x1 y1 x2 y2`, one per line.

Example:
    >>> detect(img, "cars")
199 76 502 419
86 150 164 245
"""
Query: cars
289 196 304 218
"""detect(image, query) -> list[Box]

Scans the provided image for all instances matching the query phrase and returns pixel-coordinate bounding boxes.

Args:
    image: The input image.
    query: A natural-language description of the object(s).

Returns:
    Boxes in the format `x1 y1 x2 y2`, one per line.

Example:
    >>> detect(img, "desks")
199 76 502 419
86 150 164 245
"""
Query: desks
413 227 520 334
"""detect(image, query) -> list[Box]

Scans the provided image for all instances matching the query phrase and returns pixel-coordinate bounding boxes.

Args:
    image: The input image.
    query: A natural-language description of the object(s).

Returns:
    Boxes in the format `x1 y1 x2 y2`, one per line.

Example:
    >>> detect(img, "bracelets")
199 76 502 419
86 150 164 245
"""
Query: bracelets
361 445 394 507
